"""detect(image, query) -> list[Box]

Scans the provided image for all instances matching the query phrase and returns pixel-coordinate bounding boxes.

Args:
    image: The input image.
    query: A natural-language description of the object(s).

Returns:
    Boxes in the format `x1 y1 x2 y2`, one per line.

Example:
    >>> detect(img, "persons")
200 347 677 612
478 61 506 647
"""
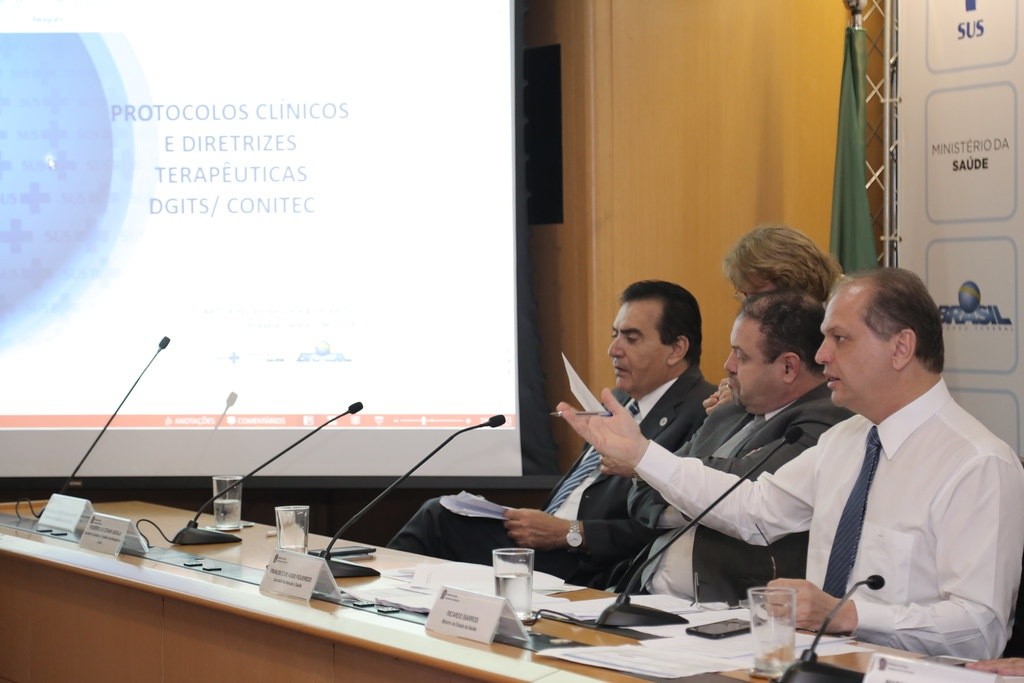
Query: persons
385 280 719 596
704 225 854 420
625 288 857 608
556 266 1024 663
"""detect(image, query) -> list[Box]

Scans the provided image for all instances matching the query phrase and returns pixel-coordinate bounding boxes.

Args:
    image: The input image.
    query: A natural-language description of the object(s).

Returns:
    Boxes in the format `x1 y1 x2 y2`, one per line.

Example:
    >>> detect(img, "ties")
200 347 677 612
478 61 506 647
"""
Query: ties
641 413 765 590
544 400 640 516
822 426 883 598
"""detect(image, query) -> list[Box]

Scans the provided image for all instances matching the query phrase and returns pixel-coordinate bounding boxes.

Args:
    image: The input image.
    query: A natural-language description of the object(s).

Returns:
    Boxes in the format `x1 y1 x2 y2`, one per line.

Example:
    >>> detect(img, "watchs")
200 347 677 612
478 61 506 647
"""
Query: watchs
566 520 583 552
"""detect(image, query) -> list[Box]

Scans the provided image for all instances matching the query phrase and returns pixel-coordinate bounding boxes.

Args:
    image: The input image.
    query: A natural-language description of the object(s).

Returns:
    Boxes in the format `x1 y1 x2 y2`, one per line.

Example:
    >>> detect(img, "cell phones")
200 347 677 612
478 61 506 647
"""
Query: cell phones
308 546 376 556
686 618 751 639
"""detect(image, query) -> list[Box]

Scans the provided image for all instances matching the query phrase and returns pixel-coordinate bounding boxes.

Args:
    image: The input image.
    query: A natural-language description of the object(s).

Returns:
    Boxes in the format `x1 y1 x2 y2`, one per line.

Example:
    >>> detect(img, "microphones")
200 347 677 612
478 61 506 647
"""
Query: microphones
36 336 170 518
598 426 804 625
172 402 364 545
780 574 885 683
321 414 506 577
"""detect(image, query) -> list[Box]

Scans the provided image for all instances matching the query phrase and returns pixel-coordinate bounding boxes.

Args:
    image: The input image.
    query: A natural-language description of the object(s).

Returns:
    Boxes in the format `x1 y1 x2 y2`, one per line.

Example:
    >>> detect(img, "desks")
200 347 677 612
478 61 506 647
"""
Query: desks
0 500 924 683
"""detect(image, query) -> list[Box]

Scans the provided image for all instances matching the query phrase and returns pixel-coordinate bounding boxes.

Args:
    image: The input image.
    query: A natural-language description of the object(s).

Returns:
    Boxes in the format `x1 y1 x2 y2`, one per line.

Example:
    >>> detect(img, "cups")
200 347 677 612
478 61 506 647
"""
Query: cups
211 474 243 532
492 548 534 621
274 505 310 556
747 587 798 679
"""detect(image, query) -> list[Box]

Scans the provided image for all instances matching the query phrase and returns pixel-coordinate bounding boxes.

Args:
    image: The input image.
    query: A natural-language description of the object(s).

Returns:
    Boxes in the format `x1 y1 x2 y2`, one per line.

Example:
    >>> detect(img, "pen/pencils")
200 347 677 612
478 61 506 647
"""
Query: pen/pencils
549 411 612 416
267 531 278 537
